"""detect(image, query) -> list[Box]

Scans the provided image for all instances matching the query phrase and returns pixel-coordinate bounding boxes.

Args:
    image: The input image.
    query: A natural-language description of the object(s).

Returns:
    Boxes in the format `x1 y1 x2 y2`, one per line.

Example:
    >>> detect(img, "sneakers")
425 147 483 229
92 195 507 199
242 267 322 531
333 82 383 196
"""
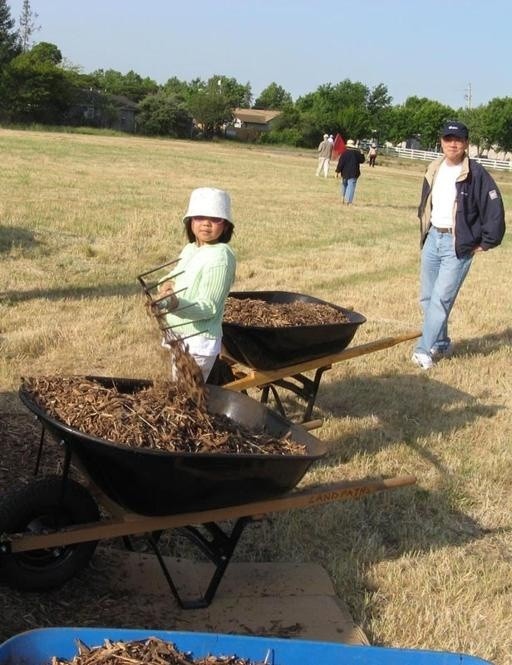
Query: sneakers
431 339 455 357
411 352 434 371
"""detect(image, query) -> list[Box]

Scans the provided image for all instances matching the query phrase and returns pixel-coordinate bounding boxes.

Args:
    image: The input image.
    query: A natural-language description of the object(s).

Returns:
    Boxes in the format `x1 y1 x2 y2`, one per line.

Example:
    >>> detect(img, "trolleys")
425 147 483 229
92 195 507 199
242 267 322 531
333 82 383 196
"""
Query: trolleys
213 291 425 423
0 377 417 611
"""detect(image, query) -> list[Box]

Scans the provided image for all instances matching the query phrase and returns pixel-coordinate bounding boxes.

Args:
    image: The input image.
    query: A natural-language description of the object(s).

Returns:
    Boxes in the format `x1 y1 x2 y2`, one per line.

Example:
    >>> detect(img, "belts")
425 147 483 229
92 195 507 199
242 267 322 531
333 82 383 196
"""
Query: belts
435 226 452 233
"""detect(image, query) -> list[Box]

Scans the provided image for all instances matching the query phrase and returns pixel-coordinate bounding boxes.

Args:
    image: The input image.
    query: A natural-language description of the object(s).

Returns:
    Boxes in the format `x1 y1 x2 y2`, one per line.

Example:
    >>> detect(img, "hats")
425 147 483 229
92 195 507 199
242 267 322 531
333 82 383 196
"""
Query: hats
345 140 356 148
182 187 234 227
441 122 469 139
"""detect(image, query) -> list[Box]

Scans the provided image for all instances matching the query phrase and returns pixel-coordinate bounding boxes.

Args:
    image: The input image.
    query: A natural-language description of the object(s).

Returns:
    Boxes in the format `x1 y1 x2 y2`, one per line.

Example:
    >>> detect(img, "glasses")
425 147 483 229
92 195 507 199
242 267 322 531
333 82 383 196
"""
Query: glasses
193 216 223 225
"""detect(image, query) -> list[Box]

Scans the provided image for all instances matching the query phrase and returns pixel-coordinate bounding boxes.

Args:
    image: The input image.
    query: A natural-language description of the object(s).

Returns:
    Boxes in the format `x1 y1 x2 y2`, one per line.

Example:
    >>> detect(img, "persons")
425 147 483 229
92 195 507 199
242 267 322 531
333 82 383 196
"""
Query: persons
335 139 366 206
318 134 332 178
368 145 376 167
158 188 236 387
408 124 505 371
328 135 334 144
